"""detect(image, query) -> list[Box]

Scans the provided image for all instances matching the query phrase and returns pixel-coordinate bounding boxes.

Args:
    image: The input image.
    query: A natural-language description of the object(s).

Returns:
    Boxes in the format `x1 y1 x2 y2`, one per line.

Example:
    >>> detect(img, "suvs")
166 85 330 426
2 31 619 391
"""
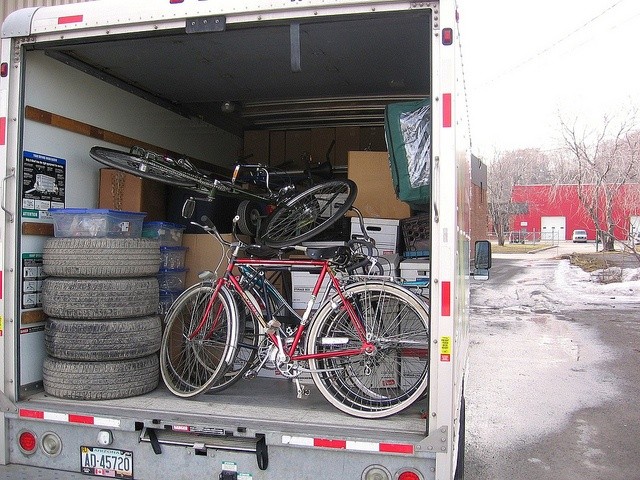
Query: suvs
508 232 526 244
572 230 587 243
633 233 640 244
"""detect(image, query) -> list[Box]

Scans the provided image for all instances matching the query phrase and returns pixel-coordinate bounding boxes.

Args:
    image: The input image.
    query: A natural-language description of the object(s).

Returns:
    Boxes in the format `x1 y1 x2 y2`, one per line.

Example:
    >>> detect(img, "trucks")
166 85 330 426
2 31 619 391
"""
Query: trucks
0 0 493 480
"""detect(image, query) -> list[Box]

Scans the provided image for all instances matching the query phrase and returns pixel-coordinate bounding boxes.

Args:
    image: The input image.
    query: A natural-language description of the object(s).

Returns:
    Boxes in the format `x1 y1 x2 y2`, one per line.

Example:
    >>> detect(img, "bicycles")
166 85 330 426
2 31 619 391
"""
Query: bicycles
87 139 359 249
190 202 430 402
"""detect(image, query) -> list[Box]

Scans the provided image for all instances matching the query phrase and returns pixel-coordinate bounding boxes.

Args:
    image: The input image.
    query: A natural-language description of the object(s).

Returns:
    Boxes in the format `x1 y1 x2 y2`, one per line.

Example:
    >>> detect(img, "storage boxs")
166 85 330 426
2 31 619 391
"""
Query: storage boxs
314 193 331 218
141 221 187 247
157 267 189 290
160 246 190 269
350 217 401 256
168 314 232 371
182 234 250 314
345 151 410 218
97 167 167 221
156 290 186 315
237 257 430 393
331 192 348 216
48 207 148 239
